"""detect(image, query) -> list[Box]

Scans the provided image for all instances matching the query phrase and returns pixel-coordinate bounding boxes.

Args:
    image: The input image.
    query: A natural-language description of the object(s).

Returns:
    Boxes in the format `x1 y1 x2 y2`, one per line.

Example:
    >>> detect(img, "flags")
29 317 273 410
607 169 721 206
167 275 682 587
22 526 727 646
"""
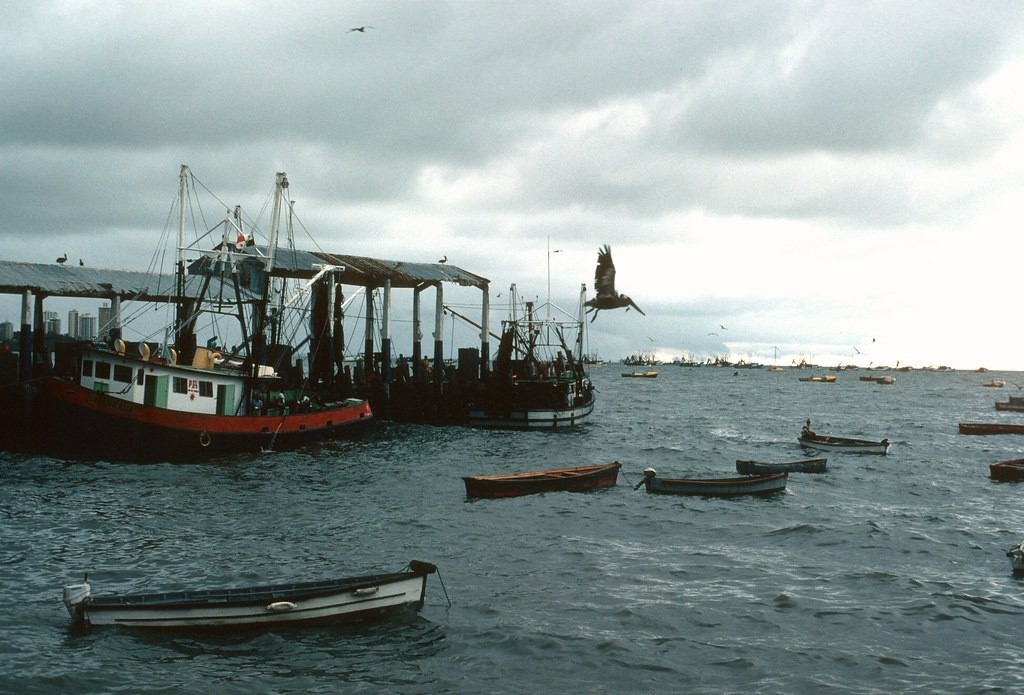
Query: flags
236 235 245 249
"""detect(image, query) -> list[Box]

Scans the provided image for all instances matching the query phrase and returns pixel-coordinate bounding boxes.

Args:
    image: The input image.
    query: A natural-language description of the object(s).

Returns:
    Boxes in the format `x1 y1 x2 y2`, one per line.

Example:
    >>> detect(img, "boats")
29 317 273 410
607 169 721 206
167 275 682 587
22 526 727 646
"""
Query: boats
642 468 788 500
369 231 599 425
734 457 829 477
995 396 1024 412
796 418 890 459
585 346 1007 390
989 459 1024 483
58 560 439 632
40 165 375 450
1005 539 1024 572
958 422 1024 437
462 462 624 501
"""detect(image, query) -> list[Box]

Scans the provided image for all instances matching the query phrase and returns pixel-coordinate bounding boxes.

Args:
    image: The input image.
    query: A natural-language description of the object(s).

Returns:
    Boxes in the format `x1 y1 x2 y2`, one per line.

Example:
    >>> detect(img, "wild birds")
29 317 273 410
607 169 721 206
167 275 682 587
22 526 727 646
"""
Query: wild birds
56 253 68 266
583 243 646 324
346 25 376 34
438 255 448 264
79 259 84 266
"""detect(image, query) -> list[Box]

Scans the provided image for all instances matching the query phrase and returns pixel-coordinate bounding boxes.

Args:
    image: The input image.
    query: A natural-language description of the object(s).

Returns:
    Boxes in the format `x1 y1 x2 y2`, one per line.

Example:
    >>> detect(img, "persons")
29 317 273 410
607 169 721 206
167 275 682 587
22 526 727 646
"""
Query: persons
278 388 286 417
253 394 263 417
396 354 429 384
